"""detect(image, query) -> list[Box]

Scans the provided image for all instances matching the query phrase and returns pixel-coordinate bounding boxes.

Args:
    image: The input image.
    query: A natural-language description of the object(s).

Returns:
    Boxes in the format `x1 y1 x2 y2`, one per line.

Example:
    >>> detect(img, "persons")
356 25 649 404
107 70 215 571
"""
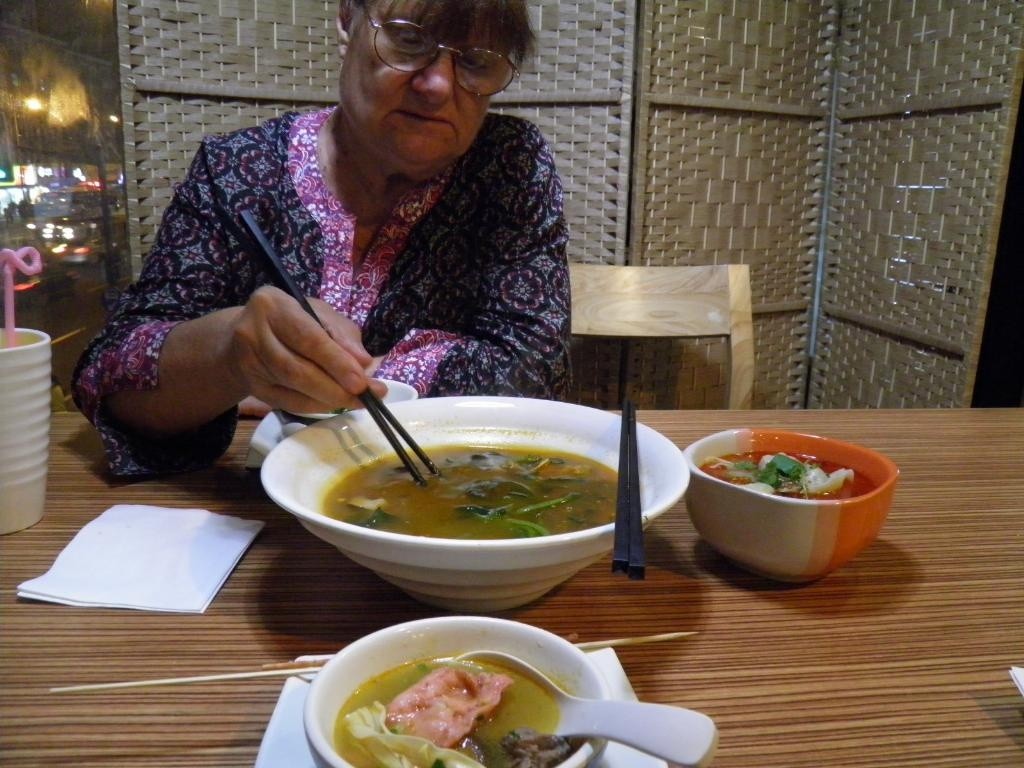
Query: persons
69 0 570 481
50 375 65 413
3 198 37 220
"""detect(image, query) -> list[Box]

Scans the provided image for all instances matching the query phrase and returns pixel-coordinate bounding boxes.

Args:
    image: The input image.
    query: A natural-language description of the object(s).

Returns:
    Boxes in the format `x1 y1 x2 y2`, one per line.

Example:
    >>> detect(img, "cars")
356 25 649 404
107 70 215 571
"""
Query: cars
0 186 122 317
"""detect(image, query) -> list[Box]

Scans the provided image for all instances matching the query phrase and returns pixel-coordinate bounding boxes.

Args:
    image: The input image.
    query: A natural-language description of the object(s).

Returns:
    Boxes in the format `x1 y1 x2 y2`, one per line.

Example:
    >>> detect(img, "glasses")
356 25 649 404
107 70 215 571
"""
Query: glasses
359 8 520 97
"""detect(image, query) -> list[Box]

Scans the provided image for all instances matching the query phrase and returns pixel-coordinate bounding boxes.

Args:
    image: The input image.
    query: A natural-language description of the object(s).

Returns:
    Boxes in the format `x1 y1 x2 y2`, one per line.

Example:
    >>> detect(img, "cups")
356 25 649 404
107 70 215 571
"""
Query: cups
0 322 56 534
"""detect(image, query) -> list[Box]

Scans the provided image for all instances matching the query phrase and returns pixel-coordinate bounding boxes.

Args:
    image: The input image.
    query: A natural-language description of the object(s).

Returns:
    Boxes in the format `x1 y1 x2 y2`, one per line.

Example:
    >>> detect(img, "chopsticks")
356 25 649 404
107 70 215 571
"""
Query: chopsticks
230 206 447 488
609 390 647 581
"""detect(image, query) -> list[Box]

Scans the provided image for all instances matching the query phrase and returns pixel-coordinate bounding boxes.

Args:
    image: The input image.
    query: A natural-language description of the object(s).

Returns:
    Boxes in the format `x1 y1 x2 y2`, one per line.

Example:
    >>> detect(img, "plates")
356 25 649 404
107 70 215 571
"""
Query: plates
248 377 423 460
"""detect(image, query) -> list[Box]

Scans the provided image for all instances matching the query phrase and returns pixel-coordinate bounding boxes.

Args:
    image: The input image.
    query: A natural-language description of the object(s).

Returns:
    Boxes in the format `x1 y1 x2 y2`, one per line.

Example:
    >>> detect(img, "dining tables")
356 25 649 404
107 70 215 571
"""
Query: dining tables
0 411 1024 767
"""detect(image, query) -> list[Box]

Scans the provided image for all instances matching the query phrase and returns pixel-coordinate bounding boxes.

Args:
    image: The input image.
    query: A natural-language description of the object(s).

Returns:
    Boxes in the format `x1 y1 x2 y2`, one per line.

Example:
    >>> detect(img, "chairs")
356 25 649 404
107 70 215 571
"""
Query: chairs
570 260 753 411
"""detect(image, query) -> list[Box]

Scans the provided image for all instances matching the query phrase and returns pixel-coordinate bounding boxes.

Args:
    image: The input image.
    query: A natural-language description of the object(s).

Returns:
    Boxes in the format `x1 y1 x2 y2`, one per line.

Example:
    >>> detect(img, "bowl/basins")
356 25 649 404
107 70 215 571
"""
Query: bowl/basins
303 617 612 768
682 425 900 585
261 394 693 616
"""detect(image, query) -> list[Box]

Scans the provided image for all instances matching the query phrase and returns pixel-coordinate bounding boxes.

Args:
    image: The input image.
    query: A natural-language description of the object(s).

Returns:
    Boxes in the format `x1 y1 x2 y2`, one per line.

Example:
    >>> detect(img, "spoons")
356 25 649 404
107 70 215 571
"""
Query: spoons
447 650 720 767
